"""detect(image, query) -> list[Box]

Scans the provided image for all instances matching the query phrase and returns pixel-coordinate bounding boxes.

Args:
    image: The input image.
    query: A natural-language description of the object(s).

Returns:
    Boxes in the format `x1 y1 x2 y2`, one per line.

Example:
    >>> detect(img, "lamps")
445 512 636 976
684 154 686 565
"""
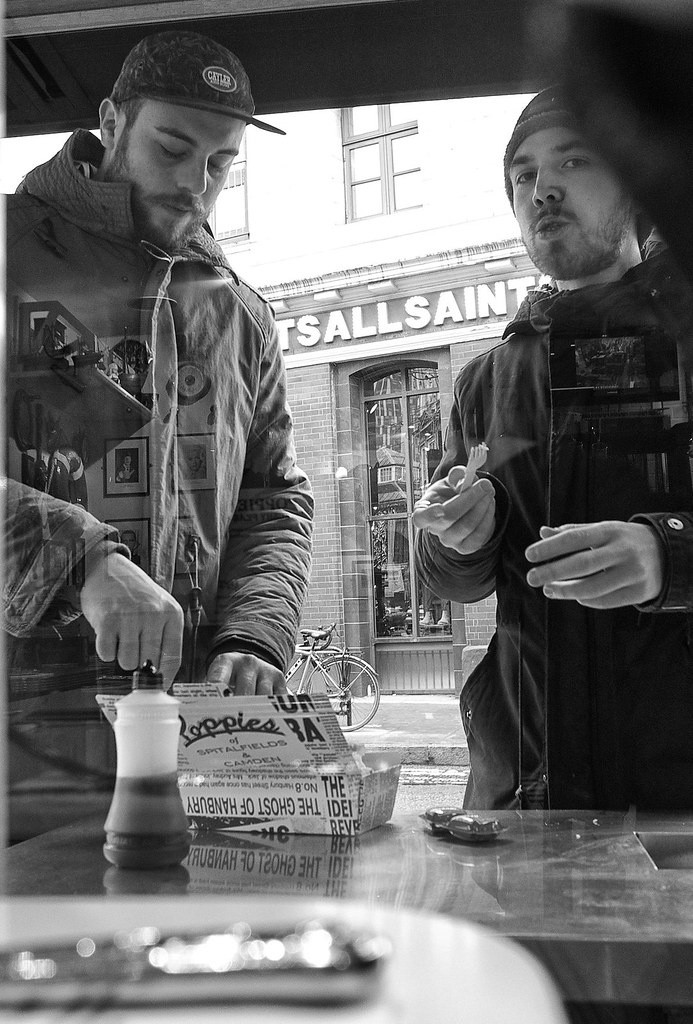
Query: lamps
368 280 397 294
485 259 515 274
312 289 343 304
271 301 289 312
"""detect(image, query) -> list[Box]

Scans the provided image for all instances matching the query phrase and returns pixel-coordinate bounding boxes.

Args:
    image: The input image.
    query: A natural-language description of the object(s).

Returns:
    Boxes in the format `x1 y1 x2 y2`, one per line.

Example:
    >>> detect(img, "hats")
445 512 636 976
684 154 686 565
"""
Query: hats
110 32 286 136
503 81 654 252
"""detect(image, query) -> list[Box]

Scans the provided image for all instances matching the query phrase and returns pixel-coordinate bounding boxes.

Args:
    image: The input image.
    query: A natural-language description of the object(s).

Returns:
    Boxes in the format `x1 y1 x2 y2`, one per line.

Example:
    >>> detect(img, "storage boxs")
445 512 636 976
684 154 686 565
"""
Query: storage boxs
93 692 402 839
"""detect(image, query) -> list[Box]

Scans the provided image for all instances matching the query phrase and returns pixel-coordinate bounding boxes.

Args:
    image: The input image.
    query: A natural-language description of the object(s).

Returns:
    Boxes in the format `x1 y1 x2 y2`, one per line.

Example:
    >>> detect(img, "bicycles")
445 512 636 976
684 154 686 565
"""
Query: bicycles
283 629 382 732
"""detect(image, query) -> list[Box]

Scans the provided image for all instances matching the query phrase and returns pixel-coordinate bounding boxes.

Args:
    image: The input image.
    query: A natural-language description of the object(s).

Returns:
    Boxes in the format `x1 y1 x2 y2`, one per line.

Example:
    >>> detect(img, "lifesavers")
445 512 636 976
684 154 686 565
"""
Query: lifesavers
48 445 89 539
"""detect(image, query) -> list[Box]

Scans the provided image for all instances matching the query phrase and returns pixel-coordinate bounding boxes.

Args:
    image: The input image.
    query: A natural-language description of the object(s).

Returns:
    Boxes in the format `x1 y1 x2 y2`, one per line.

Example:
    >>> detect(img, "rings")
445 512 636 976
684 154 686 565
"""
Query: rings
413 500 430 510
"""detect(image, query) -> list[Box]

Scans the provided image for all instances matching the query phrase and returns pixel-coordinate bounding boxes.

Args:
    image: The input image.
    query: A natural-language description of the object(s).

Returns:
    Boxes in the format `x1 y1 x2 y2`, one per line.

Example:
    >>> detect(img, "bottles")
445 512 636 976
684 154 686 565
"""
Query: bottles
102 659 191 868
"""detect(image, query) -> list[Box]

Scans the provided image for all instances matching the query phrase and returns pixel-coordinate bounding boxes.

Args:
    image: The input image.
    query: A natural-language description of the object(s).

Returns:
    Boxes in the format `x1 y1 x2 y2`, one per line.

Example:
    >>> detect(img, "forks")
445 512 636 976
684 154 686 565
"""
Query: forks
459 442 487 492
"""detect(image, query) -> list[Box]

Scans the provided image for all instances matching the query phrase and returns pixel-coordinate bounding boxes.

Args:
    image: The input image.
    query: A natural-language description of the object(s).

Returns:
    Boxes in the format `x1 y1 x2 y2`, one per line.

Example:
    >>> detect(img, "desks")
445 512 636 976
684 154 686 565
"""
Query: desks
0 812 692 1008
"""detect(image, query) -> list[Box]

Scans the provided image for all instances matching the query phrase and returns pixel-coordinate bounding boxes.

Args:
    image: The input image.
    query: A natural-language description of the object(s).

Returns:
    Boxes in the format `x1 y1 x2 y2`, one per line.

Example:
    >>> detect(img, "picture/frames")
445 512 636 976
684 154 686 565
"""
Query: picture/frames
20 451 34 484
102 516 152 579
43 539 70 588
13 388 35 450
172 430 216 494
103 437 149 498
171 514 208 577
72 538 86 584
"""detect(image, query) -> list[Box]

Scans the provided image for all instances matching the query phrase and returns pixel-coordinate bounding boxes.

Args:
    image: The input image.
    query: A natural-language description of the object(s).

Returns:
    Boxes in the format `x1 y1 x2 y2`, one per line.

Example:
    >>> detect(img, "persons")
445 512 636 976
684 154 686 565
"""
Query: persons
410 83 693 810
3 31 312 844
